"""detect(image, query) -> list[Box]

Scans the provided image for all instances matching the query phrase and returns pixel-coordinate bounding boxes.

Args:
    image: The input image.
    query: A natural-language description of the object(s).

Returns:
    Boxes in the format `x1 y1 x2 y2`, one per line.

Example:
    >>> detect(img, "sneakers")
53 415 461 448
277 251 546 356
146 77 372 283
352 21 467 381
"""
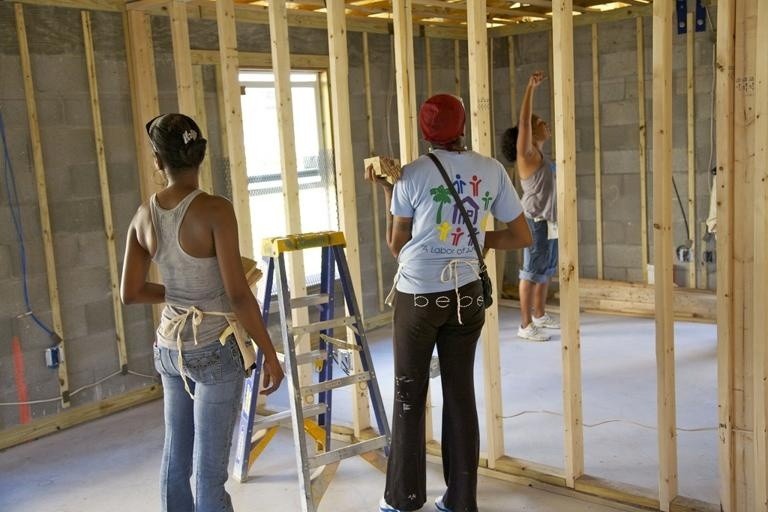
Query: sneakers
433 495 452 512
530 312 560 329
517 321 551 341
378 496 424 512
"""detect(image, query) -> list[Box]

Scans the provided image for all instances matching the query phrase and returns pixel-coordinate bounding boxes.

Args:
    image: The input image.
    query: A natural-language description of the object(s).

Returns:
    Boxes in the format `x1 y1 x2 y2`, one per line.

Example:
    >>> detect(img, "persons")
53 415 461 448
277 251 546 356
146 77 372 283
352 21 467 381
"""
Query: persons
119 113 284 512
501 70 558 341
364 94 533 512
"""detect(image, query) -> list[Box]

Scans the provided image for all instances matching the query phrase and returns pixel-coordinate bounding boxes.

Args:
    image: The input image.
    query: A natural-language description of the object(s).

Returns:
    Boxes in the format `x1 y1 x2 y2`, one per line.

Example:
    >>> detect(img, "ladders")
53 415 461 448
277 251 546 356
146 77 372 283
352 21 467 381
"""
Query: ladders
232 230 391 512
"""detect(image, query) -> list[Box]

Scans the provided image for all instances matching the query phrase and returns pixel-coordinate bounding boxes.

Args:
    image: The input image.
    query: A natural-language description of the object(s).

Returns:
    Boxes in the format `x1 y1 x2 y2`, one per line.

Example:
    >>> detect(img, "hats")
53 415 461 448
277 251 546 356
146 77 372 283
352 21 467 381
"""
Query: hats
419 93 466 144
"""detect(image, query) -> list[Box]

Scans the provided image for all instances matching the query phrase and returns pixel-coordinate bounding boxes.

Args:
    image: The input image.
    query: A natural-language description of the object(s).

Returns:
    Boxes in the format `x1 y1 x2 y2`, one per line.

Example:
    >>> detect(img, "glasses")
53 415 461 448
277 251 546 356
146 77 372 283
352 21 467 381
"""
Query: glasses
145 113 165 153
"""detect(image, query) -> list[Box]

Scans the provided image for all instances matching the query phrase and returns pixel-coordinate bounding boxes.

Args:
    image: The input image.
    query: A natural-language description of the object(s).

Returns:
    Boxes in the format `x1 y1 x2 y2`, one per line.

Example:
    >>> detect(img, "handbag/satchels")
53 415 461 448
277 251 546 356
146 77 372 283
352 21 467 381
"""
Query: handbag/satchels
480 272 494 309
